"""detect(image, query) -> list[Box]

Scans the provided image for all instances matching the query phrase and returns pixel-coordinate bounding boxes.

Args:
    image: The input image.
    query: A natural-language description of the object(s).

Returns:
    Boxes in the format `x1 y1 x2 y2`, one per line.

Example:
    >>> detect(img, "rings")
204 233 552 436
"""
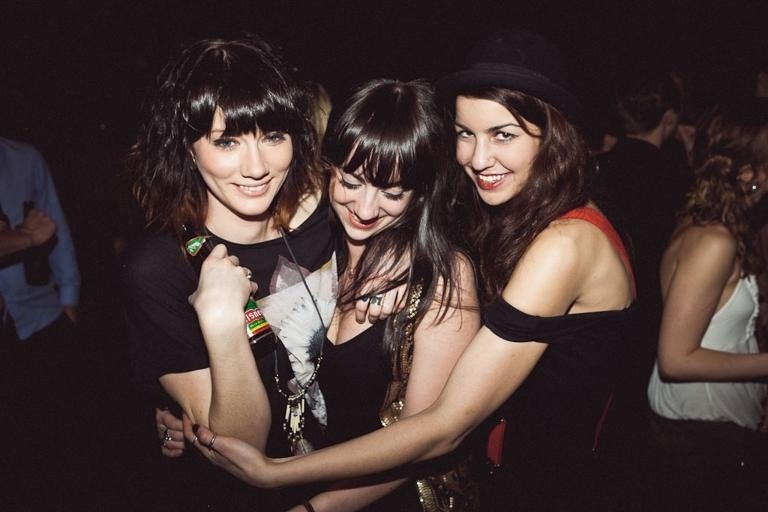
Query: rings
160 431 171 444
191 438 198 444
208 433 217 444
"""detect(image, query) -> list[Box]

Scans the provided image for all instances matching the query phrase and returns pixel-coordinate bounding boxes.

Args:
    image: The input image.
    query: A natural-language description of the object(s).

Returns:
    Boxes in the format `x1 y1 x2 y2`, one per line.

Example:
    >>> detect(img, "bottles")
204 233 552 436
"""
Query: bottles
179 221 279 361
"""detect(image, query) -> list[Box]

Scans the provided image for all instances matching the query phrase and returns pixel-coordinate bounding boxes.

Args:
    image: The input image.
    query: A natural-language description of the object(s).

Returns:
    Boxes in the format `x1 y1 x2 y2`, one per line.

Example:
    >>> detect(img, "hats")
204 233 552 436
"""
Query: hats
436 26 586 117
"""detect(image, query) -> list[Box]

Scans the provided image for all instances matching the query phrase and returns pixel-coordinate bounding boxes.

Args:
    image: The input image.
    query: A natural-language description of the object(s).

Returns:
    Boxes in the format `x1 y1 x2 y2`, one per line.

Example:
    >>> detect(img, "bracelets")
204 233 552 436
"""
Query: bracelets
301 499 313 511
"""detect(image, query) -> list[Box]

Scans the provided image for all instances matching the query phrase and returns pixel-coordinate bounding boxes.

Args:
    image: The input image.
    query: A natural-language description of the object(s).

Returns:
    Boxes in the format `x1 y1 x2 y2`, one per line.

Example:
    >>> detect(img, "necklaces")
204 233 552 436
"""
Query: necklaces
268 206 326 453
347 265 355 277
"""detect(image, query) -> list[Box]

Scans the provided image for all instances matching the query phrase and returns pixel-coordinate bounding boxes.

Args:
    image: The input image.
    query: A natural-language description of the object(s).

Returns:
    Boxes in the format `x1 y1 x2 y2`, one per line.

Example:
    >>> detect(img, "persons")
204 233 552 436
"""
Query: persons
0 206 58 264
748 60 768 99
154 42 644 511
604 69 698 328
119 36 341 510
0 134 83 507
646 92 768 512
286 77 482 511
